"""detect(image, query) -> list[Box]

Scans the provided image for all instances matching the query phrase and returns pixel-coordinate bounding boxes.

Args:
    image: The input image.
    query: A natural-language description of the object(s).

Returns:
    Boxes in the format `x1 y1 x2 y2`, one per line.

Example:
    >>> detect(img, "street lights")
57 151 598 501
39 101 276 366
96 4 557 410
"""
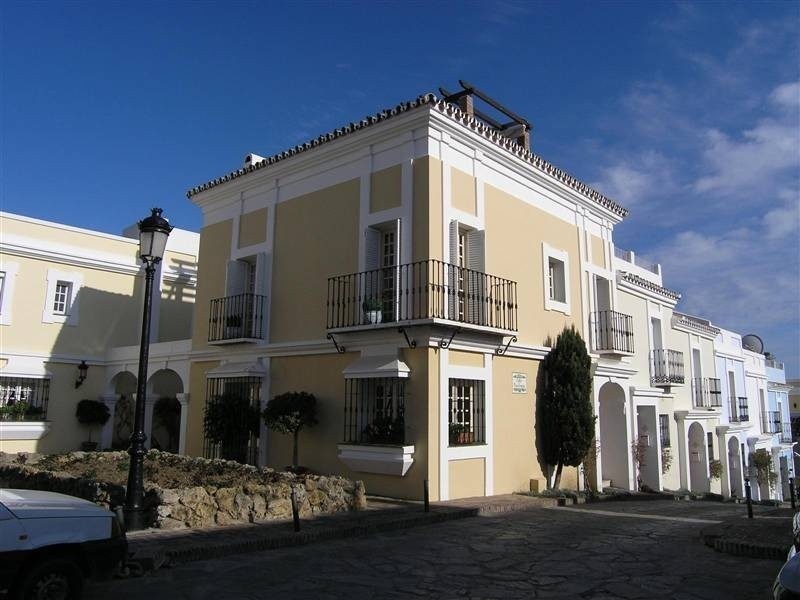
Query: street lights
109 207 177 552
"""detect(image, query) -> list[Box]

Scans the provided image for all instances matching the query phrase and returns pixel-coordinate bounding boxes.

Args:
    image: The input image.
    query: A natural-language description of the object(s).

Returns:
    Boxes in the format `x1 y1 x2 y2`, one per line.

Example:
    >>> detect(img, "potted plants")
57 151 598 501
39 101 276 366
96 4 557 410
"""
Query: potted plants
362 298 384 322
77 400 112 451
227 315 241 337
263 391 319 475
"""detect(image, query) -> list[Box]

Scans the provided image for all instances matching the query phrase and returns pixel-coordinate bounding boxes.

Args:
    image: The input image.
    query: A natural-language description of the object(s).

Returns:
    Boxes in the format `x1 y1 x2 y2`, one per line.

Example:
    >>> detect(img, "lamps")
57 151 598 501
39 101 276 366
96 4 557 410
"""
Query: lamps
75 359 89 388
